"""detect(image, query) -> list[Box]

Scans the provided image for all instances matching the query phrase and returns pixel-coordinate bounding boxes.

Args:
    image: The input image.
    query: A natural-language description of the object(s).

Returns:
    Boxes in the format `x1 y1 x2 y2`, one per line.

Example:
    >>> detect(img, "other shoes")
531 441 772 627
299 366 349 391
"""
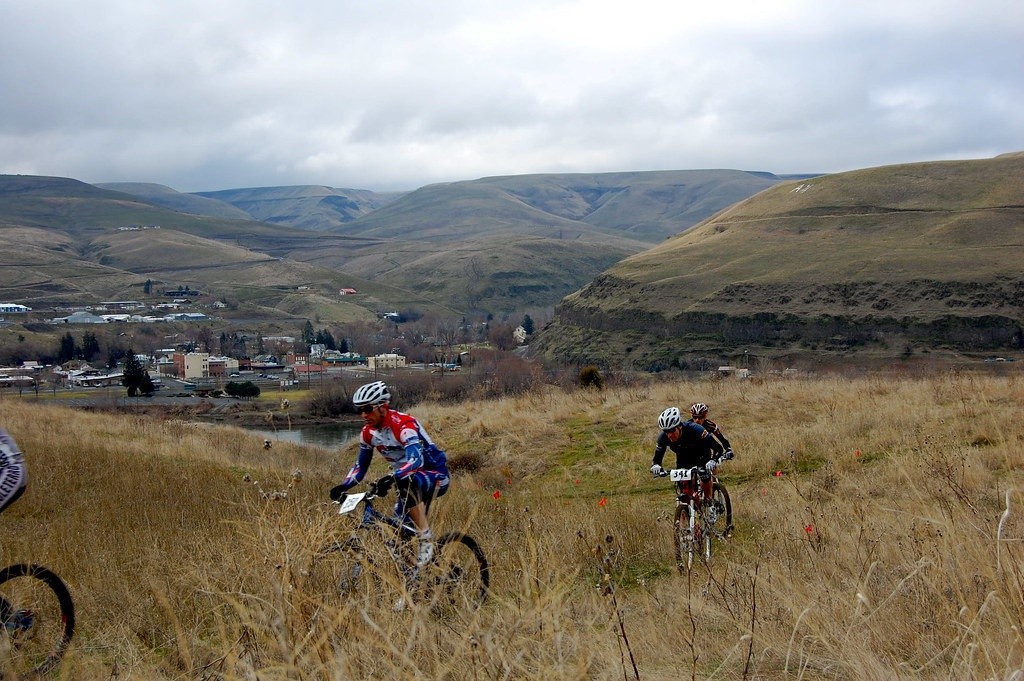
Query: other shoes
704 501 718 523
416 529 435 566
391 595 411 612
11 609 36 650
679 531 691 550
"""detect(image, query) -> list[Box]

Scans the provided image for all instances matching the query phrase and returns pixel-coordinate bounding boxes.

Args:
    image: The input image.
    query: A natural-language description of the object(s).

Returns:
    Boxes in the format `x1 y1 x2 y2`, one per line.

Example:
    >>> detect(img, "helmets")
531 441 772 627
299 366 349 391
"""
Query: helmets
690 403 709 417
352 380 392 407
657 406 681 430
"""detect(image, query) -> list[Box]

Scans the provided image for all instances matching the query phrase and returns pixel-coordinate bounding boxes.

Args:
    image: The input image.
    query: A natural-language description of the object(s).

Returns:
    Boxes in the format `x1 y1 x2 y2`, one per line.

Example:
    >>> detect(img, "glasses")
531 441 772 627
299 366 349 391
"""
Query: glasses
662 427 678 434
354 405 379 415
693 416 703 419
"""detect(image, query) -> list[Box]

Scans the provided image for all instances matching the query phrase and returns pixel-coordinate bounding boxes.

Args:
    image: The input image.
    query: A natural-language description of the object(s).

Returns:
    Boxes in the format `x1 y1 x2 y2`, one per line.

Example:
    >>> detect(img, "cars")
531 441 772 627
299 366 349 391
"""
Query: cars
258 373 280 380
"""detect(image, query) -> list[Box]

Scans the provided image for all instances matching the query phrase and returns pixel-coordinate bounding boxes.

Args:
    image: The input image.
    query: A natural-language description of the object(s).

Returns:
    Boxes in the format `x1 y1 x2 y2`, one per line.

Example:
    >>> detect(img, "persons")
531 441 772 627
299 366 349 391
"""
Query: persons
651 408 723 541
686 404 734 511
0 425 32 639
331 381 450 611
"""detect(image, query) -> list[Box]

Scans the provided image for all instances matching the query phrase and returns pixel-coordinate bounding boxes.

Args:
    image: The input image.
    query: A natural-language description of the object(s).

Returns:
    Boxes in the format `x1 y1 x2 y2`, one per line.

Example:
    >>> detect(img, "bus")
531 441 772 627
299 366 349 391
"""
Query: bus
184 384 198 390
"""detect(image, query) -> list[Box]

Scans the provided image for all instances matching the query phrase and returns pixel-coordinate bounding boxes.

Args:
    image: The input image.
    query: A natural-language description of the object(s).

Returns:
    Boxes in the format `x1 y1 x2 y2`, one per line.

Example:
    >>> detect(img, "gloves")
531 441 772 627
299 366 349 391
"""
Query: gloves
650 464 661 475
377 476 393 497
330 485 345 501
725 450 734 460
706 460 716 475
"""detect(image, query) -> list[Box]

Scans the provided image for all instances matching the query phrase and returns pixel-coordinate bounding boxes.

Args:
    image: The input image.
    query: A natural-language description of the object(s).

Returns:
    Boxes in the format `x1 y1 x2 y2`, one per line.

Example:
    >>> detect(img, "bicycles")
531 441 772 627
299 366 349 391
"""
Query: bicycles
0 564 78 681
648 464 720 575
703 452 737 538
305 473 492 611
